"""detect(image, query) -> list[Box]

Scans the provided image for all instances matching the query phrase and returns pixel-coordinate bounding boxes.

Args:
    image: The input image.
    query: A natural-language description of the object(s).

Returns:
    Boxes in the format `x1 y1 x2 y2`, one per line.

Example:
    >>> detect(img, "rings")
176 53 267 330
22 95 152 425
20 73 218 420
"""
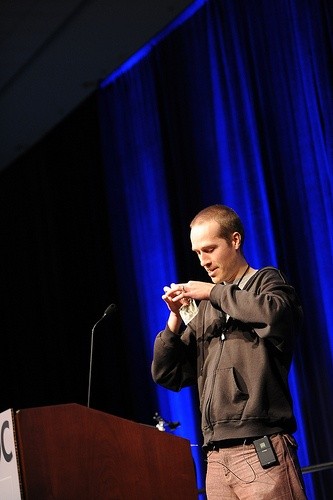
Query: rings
182 285 185 291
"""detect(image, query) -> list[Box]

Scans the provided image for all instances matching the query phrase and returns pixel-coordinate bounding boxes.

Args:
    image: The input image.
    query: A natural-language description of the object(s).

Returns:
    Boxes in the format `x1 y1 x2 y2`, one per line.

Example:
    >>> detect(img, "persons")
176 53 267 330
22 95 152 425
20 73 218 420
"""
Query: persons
152 205 310 500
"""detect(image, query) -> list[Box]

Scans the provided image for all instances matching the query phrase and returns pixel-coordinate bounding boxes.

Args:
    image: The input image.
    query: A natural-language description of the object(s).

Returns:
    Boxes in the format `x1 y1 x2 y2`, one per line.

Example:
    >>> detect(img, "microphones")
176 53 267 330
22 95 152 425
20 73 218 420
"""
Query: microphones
87 303 115 408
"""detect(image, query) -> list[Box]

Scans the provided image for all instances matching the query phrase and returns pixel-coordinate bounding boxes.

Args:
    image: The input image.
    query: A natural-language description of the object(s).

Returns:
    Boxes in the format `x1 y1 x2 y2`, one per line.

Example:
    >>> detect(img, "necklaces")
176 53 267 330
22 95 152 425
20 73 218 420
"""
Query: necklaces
235 266 250 286
